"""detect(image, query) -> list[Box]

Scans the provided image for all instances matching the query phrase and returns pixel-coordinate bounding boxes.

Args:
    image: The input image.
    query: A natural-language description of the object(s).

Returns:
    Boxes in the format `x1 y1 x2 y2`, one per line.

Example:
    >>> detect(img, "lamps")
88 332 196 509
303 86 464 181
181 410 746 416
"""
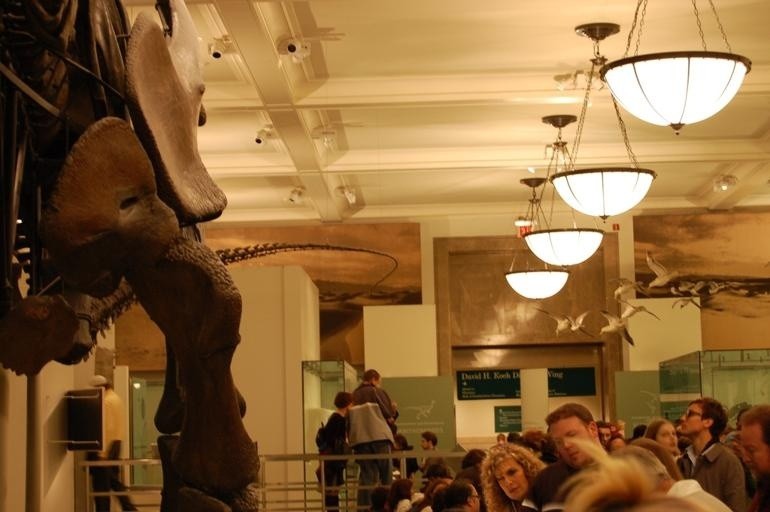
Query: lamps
549 22 660 224
503 176 571 300
601 0 751 130
522 115 604 267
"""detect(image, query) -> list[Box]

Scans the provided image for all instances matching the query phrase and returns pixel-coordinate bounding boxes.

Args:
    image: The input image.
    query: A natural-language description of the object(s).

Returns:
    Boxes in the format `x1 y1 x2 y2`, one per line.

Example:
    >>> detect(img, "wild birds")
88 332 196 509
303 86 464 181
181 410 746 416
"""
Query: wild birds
535 305 587 338
598 247 729 346
560 310 595 338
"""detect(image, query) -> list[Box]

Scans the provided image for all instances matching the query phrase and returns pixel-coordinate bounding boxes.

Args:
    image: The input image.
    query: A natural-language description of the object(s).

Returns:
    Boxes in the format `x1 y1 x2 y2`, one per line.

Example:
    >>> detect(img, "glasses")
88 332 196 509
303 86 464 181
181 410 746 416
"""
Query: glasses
686 409 701 417
473 495 481 499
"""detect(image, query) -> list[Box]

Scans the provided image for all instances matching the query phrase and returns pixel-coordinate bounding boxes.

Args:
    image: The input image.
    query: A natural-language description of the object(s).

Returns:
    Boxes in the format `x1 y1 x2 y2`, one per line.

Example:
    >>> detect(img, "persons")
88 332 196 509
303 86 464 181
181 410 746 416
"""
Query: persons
375 397 770 511
316 371 419 511
87 375 137 511
